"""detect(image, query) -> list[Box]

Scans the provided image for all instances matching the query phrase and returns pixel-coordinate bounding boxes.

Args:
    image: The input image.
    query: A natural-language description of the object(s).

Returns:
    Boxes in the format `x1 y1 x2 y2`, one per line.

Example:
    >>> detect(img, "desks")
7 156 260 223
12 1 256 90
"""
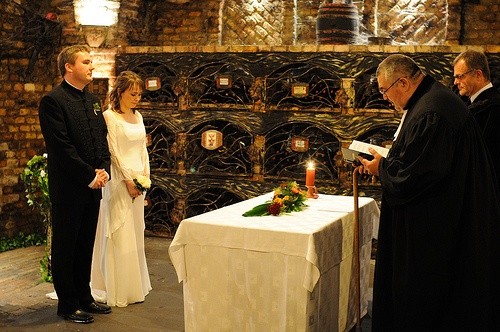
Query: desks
168 192 380 332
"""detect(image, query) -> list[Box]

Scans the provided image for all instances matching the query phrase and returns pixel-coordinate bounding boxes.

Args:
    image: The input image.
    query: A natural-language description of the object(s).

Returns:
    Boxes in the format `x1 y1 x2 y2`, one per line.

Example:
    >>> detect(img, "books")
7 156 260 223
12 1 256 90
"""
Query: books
342 140 391 169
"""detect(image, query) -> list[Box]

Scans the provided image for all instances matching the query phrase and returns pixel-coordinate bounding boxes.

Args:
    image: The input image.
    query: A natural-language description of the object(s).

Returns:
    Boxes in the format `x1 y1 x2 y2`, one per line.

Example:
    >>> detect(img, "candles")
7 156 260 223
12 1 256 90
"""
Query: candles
306 160 315 186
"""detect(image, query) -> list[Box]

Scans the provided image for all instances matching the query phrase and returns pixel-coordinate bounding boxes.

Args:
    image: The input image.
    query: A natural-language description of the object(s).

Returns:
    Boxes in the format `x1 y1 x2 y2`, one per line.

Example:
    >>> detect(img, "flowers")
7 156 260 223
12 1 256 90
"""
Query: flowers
242 179 308 218
132 176 151 204
21 152 54 209
93 103 101 115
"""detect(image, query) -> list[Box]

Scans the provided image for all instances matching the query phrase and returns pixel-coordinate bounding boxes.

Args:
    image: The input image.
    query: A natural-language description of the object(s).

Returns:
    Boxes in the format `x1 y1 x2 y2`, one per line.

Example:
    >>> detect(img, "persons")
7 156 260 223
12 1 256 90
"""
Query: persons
355 49 500 332
35 46 151 324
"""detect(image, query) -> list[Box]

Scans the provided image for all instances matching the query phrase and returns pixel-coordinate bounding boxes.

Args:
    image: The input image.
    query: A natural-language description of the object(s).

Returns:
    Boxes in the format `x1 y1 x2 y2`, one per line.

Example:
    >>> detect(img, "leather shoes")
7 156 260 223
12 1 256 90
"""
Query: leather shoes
81 300 112 314
57 309 95 324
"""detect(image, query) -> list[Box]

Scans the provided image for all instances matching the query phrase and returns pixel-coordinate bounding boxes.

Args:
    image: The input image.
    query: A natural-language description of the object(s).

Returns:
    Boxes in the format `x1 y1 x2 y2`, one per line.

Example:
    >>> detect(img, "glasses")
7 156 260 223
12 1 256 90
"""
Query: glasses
379 76 407 95
453 67 483 82
